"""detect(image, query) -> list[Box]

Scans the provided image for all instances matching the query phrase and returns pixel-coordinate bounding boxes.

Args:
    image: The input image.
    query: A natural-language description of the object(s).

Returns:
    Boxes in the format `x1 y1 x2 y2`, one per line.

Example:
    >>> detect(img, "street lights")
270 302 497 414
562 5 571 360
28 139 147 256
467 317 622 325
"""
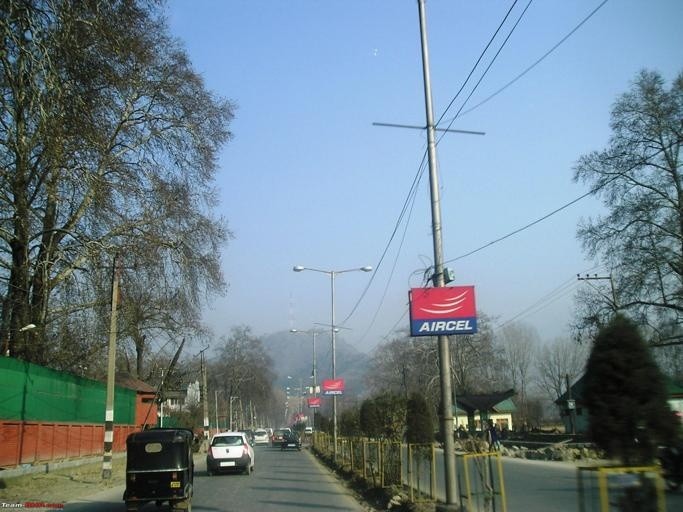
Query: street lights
284 265 373 460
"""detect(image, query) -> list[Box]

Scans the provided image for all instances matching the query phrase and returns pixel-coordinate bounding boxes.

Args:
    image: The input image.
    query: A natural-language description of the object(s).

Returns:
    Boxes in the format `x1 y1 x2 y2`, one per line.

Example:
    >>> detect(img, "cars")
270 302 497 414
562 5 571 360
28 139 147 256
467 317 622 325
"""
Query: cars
123 428 201 512
207 427 312 476
